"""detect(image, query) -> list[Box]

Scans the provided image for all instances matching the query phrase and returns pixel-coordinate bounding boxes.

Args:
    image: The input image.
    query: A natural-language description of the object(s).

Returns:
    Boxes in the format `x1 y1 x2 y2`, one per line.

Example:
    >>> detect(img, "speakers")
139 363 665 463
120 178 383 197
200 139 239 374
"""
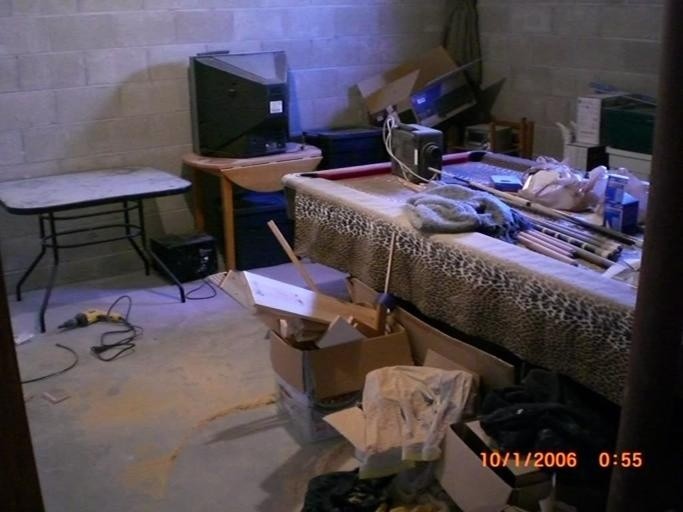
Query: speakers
150 228 218 286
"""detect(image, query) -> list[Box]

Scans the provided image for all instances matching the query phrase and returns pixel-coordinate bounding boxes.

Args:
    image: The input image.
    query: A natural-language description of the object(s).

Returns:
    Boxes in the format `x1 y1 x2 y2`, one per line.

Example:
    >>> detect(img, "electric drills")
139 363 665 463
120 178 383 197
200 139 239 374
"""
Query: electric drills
56 308 124 329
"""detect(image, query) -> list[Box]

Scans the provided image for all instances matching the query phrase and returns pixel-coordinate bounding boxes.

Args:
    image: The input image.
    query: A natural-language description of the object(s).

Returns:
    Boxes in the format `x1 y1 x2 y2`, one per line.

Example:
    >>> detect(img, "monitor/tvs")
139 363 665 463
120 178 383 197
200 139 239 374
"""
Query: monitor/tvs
190 50 290 158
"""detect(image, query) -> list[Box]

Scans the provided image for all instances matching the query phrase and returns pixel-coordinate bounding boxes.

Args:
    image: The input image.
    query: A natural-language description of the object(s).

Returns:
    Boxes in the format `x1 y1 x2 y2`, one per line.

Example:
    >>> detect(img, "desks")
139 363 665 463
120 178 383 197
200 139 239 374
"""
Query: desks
181 142 323 275
0 165 193 333
281 148 653 409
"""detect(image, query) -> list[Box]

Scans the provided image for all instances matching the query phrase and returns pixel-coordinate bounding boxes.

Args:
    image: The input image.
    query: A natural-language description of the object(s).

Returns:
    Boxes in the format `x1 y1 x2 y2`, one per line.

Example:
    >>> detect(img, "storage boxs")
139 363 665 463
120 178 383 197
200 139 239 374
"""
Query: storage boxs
355 44 484 129
268 318 408 444
431 414 560 512
561 94 655 181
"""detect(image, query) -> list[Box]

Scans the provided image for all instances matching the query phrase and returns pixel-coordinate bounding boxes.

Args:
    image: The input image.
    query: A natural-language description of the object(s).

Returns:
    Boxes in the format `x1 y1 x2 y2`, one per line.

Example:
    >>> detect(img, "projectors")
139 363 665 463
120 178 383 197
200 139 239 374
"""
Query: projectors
392 121 443 182
